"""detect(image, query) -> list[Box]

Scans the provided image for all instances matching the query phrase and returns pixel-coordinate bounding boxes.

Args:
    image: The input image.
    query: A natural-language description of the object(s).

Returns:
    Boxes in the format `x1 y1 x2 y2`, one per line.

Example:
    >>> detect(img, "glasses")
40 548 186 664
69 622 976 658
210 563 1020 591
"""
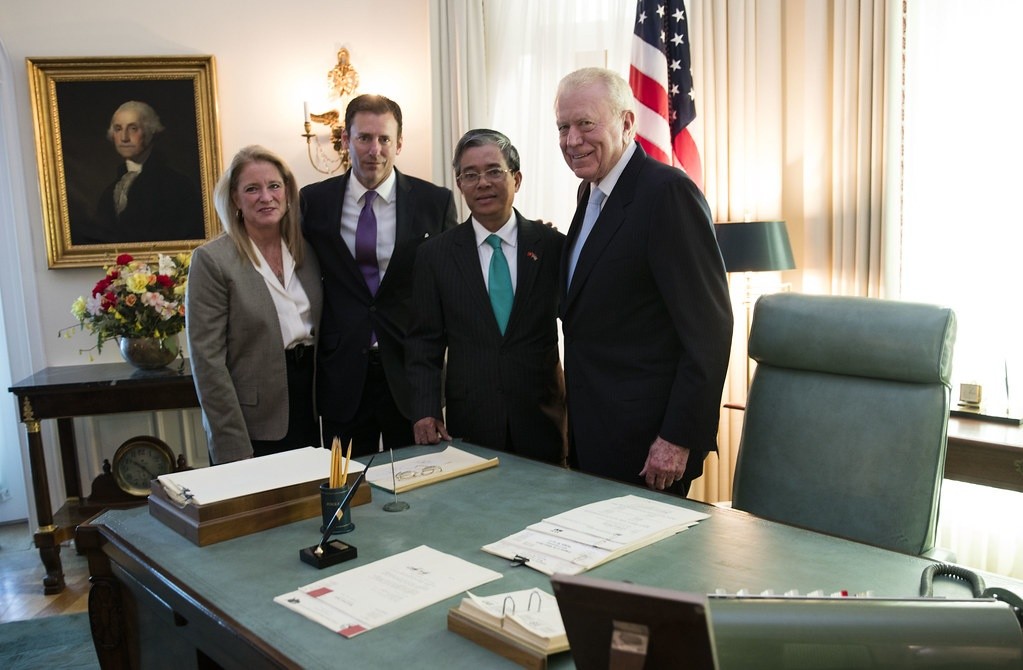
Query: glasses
458 168 514 187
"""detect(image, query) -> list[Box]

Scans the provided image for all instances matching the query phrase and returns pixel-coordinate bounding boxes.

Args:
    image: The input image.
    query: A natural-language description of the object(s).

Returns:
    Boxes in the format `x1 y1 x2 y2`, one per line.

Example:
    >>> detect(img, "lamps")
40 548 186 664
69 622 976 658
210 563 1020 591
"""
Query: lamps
712 220 796 394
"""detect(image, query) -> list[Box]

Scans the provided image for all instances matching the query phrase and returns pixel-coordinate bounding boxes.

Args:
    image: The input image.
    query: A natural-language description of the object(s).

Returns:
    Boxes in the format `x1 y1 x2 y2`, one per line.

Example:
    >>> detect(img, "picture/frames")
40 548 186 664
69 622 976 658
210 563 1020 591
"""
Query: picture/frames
25 53 223 270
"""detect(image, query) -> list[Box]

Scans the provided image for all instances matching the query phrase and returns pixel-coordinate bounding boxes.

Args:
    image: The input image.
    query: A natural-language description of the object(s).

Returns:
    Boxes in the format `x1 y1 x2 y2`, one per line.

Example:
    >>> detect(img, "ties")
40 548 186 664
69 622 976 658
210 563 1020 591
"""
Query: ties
485 235 513 337
355 191 380 346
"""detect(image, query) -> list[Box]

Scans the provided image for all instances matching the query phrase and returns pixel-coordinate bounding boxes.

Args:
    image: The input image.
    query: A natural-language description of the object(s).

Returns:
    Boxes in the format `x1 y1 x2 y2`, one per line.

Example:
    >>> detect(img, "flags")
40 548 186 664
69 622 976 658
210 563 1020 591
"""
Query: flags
628 0 703 194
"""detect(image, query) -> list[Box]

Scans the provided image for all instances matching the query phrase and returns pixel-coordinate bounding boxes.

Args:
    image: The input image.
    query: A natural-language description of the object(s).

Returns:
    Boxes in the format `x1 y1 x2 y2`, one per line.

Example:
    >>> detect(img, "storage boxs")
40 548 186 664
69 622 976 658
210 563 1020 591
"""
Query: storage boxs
148 471 371 549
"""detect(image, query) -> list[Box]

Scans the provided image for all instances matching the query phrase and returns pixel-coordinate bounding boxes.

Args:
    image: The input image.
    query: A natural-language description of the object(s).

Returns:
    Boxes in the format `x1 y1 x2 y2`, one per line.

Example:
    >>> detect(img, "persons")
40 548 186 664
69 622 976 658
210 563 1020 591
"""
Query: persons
188 147 322 467
555 67 733 499
297 92 559 460
410 128 567 469
93 101 193 244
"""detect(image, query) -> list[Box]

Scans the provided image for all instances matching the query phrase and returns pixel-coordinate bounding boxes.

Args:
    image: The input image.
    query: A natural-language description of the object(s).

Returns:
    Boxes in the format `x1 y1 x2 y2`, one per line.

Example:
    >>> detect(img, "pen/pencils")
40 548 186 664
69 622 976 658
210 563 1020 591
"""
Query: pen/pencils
329 435 354 490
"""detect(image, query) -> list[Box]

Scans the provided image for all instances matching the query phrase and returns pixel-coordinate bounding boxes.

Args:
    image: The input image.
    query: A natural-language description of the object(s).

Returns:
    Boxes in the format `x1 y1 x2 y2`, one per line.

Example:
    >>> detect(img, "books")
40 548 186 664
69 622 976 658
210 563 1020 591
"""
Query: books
362 444 500 495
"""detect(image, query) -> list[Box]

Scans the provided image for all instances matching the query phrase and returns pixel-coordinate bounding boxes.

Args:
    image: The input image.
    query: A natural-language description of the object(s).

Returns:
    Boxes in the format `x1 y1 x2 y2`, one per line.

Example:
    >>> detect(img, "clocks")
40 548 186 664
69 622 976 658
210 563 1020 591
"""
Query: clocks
957 384 981 409
87 437 196 503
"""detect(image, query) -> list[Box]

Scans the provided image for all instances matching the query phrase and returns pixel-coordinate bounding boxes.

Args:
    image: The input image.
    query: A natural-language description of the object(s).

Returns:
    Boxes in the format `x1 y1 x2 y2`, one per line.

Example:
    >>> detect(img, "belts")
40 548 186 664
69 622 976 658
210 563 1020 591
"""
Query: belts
367 351 382 367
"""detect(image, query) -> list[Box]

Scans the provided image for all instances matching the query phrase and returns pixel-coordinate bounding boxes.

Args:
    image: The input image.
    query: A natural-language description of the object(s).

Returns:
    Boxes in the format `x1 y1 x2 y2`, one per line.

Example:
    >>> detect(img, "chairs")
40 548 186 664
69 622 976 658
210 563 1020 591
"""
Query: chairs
731 294 960 562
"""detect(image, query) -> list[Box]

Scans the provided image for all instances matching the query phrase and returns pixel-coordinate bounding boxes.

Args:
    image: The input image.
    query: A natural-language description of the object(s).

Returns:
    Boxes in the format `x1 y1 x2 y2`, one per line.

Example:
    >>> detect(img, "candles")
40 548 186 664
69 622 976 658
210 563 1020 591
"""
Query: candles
303 101 312 123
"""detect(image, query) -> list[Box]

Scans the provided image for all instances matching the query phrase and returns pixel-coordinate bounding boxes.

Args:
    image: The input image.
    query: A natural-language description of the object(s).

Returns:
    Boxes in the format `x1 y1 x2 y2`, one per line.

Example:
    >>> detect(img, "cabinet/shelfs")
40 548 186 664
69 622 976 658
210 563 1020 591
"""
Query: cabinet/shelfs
8 358 213 595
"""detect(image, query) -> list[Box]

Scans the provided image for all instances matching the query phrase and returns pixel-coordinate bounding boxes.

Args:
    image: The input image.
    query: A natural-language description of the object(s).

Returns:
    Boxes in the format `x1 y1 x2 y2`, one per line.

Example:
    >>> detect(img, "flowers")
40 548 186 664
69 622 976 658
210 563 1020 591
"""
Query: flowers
56 246 194 362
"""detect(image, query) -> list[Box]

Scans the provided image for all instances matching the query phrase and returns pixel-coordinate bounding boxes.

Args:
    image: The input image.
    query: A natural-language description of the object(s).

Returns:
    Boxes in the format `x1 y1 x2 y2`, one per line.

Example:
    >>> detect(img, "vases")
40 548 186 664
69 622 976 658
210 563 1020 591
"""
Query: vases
118 333 179 370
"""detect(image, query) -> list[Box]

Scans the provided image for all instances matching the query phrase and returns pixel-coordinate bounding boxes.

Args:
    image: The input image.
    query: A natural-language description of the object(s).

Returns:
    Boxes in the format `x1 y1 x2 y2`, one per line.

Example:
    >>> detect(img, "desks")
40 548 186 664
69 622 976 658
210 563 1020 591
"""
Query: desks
72 435 1023 670
723 396 1023 495
